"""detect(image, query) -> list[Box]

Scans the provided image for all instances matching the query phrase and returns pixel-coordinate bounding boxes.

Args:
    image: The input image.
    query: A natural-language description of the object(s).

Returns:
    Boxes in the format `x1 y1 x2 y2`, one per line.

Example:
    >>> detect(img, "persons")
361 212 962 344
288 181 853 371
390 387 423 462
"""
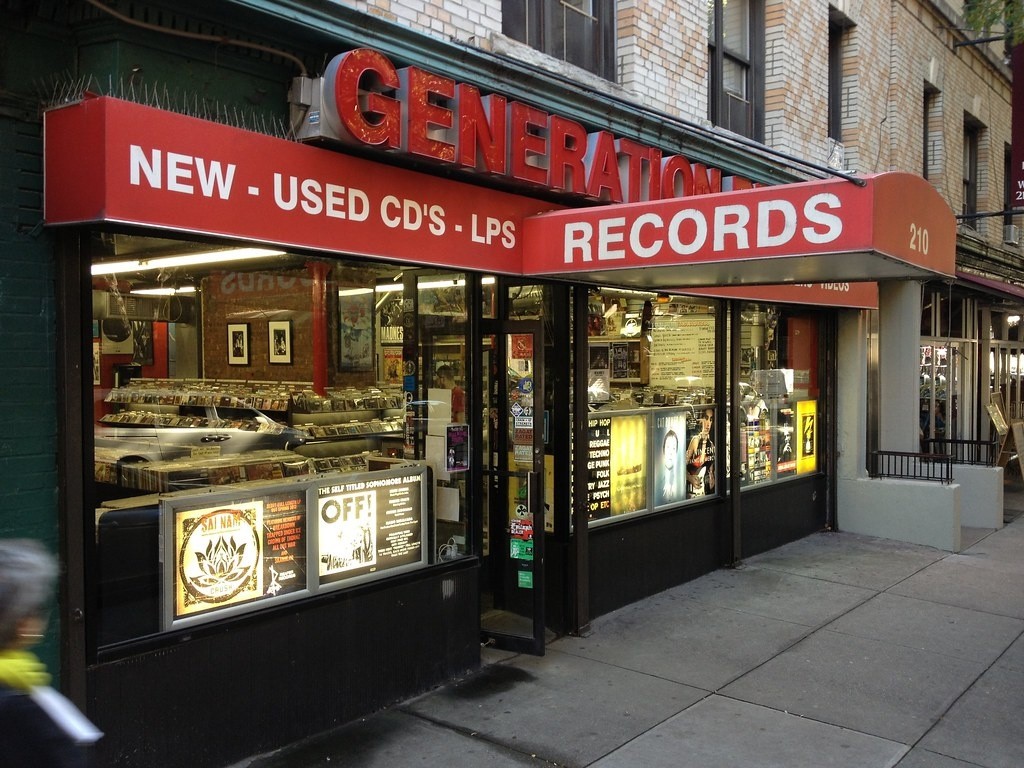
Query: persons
0 538 105 768
274 334 286 355
777 417 813 467
233 334 242 357
437 365 465 423
658 408 716 501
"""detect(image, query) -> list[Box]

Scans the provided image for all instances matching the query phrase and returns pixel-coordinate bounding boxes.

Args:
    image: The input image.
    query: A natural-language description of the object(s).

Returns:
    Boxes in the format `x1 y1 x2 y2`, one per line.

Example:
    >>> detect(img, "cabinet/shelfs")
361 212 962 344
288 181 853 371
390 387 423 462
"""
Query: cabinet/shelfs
97 397 404 457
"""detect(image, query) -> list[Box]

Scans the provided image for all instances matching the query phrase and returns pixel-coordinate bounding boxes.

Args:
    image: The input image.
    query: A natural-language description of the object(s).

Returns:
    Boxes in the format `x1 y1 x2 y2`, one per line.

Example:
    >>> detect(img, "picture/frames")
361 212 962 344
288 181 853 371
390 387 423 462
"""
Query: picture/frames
226 322 251 366
267 319 293 365
